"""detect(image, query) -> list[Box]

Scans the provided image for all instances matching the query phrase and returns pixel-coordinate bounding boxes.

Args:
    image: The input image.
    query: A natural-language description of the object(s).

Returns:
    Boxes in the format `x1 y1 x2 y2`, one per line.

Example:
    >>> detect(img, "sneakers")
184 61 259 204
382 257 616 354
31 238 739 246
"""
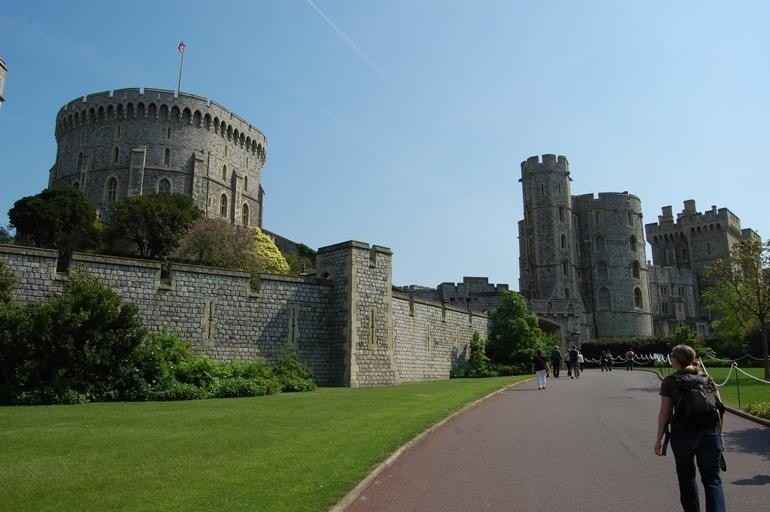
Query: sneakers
538 369 633 390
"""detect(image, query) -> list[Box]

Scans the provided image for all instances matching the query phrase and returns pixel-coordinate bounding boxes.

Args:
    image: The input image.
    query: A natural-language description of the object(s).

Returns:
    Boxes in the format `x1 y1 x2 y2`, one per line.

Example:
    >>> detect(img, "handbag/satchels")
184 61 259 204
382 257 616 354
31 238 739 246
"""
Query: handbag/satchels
564 358 567 363
542 361 549 369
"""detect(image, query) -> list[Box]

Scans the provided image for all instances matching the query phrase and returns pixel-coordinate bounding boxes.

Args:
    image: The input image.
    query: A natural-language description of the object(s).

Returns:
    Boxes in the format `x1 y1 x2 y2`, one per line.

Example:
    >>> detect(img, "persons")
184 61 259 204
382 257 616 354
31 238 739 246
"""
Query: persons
534 345 612 390
655 344 728 511
626 347 635 371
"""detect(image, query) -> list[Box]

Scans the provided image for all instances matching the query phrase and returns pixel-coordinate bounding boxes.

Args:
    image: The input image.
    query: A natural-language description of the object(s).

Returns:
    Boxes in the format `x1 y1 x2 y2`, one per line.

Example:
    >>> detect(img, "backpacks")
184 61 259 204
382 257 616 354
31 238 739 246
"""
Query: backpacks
628 351 635 359
670 371 719 442
